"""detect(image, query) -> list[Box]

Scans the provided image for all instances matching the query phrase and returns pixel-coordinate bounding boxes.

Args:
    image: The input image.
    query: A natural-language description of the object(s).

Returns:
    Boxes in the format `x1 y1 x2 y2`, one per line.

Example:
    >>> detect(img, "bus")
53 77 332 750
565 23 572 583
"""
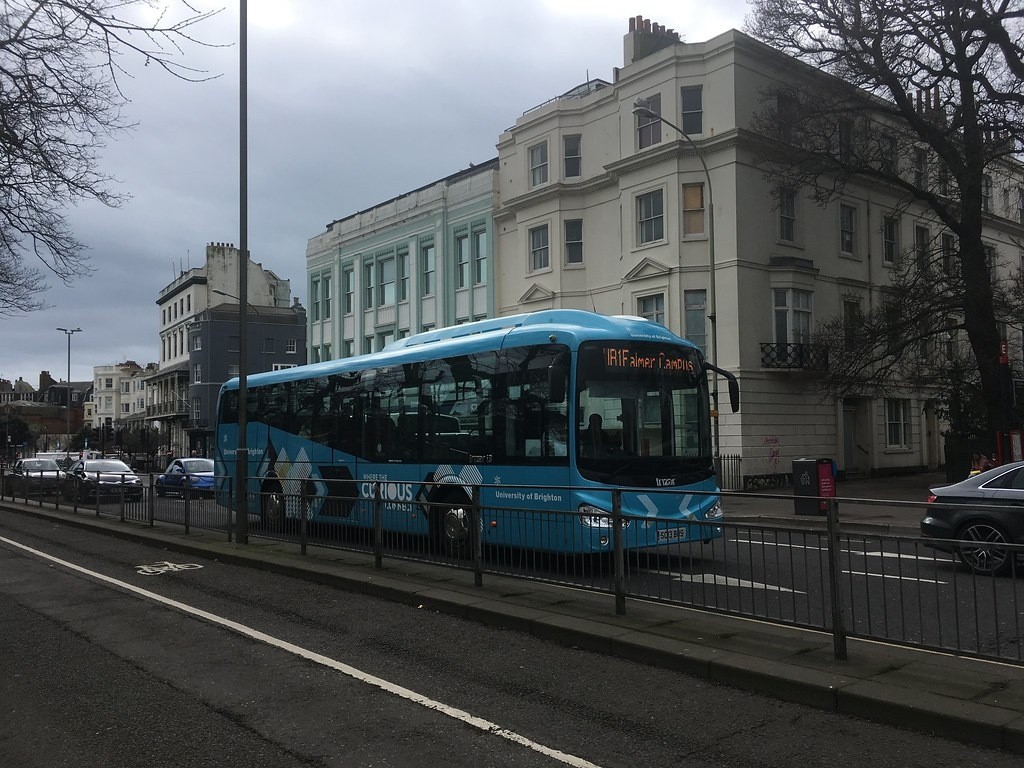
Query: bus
212 307 741 560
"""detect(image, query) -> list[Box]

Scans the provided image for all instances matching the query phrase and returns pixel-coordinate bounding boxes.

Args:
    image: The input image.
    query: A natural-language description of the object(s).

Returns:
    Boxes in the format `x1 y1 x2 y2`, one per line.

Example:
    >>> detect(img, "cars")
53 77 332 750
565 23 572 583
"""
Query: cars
920 461 1024 573
65 459 142 506
154 457 214 498
13 459 66 495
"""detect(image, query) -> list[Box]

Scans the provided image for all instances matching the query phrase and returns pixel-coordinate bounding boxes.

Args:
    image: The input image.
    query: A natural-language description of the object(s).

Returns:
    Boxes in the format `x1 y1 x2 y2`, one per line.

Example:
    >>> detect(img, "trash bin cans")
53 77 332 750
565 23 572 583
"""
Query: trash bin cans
791 457 837 516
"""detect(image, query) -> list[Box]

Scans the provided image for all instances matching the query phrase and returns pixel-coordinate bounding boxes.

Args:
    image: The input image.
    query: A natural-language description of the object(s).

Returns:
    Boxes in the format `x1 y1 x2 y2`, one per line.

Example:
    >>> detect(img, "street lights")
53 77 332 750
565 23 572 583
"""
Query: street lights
631 107 723 494
56 327 82 454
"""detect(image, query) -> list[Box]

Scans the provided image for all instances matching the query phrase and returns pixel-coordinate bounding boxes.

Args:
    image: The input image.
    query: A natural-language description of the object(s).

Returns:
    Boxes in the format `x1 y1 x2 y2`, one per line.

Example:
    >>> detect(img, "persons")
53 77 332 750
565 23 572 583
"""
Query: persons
578 413 616 460
165 450 175 468
190 449 199 457
963 453 1001 478
